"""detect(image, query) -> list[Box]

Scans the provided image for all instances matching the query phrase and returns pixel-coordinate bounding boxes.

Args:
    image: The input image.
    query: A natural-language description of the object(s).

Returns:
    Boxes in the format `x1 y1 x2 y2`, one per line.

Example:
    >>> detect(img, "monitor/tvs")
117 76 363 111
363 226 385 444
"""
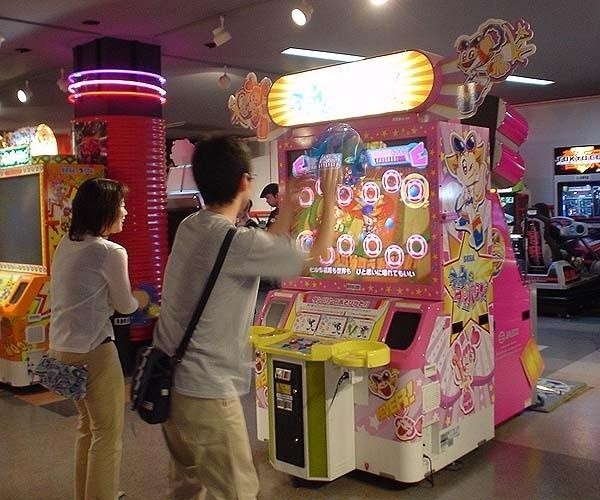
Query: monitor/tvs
0 171 43 264
553 173 600 228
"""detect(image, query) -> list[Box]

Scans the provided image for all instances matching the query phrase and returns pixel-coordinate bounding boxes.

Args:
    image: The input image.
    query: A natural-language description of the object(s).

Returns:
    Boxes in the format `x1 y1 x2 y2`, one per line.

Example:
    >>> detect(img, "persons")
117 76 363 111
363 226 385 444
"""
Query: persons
148 136 343 499
260 183 279 226
46 177 152 500
533 201 583 270
234 199 259 228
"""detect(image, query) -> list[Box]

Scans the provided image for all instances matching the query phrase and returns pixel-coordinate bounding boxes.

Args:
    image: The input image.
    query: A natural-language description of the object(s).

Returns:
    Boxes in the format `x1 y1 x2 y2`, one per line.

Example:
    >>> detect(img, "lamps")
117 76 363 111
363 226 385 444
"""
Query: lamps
290 1 313 27
217 69 229 90
17 60 70 104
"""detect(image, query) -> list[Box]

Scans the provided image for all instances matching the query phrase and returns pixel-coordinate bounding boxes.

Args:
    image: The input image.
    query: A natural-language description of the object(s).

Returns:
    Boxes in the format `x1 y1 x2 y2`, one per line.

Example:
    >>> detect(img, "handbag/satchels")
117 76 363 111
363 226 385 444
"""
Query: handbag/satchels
129 346 177 425
30 356 88 401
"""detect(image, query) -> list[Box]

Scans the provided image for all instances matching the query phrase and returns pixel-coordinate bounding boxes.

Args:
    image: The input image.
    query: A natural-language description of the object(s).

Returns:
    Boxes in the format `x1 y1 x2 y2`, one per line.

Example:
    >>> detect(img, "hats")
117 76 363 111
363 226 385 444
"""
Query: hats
260 183 278 198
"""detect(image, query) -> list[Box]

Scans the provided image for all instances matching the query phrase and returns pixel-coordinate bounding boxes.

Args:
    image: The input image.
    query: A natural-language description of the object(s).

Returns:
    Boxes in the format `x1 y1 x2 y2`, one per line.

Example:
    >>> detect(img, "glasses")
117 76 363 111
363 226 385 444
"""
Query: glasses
247 172 257 181
